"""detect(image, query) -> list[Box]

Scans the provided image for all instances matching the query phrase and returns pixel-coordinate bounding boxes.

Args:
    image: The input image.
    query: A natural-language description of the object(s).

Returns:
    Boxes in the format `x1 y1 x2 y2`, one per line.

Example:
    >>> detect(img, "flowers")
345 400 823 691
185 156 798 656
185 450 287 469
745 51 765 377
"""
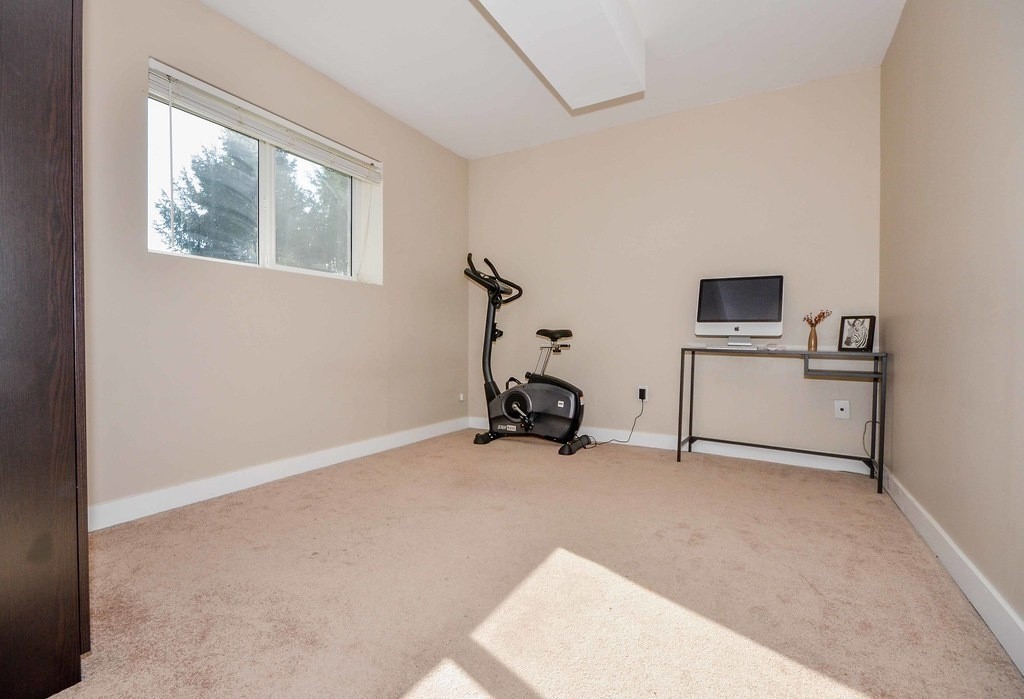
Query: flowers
804 309 832 327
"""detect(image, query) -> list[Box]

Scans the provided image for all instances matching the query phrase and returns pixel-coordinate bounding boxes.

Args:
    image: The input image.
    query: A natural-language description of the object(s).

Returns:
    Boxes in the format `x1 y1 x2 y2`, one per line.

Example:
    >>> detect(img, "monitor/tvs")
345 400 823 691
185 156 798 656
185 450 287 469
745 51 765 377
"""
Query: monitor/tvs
693 274 786 352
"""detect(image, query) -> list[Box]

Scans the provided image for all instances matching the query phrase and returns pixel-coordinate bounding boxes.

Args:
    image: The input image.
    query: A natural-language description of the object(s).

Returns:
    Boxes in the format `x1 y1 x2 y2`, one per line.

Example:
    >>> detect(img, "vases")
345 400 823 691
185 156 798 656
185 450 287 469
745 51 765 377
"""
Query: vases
807 327 816 352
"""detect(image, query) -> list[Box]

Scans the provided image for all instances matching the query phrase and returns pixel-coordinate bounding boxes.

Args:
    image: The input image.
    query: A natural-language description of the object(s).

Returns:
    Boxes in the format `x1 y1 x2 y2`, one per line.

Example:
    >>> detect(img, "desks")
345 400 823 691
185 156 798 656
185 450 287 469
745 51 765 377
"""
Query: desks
677 347 887 495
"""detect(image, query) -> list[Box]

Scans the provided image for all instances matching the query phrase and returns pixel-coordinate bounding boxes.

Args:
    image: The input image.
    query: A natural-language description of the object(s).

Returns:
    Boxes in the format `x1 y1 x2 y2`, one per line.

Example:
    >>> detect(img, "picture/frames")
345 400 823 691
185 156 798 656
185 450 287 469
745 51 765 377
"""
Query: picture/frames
837 315 876 352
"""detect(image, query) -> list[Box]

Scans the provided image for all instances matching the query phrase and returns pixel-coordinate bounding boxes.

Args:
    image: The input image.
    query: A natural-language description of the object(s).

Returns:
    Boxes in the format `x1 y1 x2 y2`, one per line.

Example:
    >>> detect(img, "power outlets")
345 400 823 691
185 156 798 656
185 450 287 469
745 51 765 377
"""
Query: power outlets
834 400 851 420
638 386 648 402
